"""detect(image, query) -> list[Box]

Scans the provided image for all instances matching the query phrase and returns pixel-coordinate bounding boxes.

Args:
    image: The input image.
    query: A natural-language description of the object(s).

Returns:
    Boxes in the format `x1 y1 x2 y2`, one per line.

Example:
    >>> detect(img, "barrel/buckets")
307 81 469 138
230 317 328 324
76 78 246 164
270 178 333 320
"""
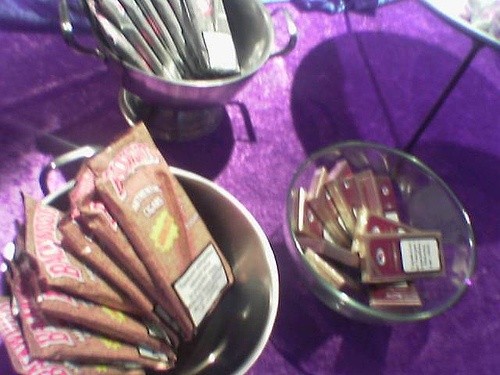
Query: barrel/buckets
59 0 297 142
40 144 279 375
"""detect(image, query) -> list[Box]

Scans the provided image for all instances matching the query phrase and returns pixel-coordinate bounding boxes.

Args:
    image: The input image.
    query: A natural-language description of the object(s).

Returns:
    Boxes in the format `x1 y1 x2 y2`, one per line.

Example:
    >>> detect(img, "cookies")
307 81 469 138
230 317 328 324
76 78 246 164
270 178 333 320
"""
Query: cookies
292 158 445 312
90 0 241 80
0 120 235 375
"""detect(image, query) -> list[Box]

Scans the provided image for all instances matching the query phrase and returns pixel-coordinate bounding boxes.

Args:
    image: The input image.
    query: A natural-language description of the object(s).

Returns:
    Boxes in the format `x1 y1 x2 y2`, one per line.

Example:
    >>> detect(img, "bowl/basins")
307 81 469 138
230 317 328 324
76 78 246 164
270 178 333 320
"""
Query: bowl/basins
283 140 475 324
